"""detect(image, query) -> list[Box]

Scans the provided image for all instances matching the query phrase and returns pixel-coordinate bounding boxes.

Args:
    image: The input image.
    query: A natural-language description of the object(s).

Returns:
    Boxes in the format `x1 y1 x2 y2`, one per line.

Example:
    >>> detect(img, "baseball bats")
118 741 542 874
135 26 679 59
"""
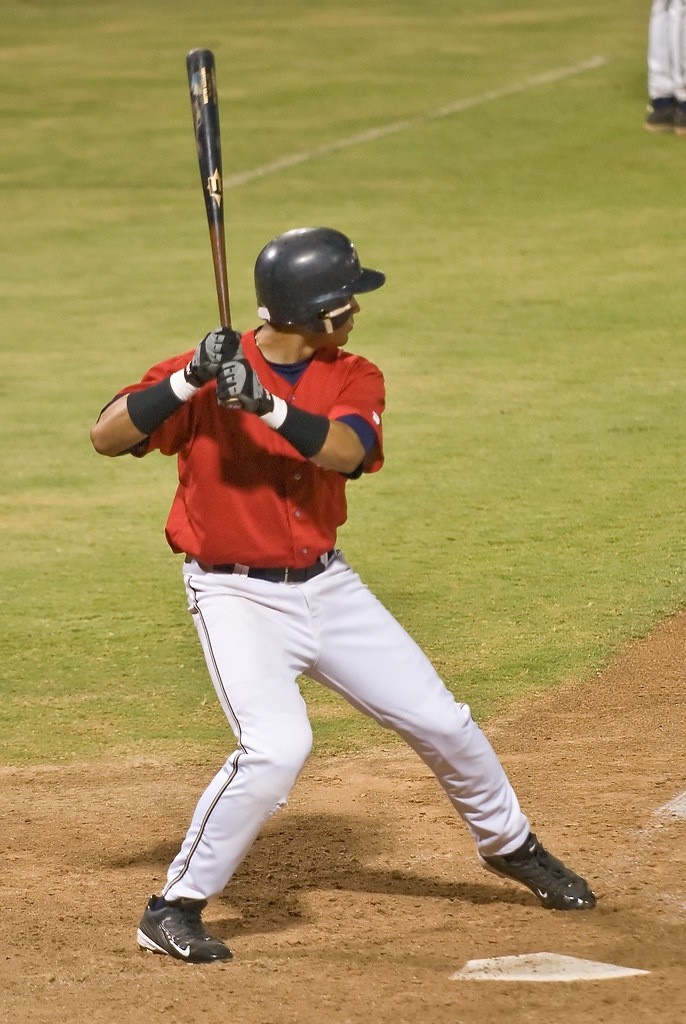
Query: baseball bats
185 48 243 410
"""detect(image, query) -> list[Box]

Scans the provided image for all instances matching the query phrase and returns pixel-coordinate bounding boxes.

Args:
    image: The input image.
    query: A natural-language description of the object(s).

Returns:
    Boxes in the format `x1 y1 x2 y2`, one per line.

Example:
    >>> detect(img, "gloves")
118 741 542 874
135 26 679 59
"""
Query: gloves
185 327 241 389
216 353 275 417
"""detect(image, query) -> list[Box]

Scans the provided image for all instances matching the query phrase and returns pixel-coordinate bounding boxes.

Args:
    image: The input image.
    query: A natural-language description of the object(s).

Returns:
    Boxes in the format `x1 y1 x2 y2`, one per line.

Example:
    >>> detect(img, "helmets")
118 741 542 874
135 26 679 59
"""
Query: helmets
255 227 386 332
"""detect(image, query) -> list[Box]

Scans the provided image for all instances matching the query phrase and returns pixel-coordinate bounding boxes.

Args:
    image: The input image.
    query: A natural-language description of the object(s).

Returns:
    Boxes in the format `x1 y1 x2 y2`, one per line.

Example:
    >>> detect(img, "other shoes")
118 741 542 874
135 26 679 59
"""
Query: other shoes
643 100 686 136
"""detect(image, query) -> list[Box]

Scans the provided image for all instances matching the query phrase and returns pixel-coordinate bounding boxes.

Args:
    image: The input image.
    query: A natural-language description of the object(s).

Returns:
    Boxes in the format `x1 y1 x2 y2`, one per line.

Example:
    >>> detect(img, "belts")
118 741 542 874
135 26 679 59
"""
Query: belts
214 550 334 585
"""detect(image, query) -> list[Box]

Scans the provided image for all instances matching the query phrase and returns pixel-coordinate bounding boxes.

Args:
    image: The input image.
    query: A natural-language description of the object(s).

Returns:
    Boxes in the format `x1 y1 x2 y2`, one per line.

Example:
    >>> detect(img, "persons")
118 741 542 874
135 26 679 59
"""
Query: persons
91 229 597 963
646 1 686 135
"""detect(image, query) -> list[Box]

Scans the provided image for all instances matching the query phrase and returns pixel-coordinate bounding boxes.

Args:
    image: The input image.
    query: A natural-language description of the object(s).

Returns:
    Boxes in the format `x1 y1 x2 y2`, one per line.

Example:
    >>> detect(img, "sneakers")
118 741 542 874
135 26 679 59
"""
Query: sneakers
137 894 233 963
477 833 596 910
